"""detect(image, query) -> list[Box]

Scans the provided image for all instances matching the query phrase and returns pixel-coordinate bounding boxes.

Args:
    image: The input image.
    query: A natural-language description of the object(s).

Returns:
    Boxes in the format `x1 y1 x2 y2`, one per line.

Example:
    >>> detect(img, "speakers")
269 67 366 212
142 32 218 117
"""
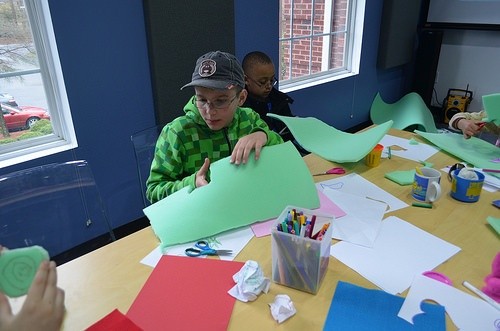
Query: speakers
443 94 467 124
404 28 443 108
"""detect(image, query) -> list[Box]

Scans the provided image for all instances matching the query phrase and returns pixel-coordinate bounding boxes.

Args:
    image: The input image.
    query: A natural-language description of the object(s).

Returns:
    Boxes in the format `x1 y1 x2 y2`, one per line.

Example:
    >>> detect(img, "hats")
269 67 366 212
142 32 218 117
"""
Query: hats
180 51 245 89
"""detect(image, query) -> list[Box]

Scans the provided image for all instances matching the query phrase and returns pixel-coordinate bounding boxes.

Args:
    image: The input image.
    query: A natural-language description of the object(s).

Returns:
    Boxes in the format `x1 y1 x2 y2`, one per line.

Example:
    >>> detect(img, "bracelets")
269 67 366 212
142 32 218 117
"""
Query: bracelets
454 117 467 130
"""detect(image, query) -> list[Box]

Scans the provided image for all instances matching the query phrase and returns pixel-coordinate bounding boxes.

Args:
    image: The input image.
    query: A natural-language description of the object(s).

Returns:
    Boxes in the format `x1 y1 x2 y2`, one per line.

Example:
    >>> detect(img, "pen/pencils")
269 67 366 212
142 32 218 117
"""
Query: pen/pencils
478 168 500 173
276 208 317 238
311 222 330 241
463 281 500 311
388 146 391 159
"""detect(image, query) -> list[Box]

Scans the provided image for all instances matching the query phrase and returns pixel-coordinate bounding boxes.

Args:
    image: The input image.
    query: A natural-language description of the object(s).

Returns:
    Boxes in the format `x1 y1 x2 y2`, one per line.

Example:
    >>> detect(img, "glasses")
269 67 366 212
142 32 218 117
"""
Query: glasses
194 90 242 109
244 72 278 88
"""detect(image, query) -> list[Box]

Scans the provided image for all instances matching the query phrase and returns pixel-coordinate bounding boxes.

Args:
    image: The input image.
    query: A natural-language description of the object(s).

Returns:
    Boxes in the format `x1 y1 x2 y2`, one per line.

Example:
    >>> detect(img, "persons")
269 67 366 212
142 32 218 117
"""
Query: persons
0 245 65 331
449 110 500 139
144 51 284 203
239 52 295 141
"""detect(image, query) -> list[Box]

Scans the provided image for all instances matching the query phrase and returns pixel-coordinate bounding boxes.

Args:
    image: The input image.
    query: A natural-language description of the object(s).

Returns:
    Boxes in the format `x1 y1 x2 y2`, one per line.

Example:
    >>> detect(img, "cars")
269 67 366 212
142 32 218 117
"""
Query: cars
0 93 50 132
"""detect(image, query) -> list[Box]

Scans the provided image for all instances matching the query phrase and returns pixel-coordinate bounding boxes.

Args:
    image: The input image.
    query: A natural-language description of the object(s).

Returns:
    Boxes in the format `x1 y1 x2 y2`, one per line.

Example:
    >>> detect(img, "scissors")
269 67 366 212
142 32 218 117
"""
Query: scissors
278 126 290 136
477 118 496 131
184 240 233 257
312 167 345 176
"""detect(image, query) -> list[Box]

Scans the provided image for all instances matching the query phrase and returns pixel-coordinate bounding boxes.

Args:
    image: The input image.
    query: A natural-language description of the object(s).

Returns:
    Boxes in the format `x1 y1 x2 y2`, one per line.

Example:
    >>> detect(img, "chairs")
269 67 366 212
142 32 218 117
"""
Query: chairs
130 124 164 207
0 159 116 257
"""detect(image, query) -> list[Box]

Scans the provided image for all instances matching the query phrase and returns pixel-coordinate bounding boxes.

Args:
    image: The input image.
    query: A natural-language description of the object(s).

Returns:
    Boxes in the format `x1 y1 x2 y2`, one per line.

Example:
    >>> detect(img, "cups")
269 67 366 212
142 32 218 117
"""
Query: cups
411 167 442 204
364 144 384 166
450 169 485 202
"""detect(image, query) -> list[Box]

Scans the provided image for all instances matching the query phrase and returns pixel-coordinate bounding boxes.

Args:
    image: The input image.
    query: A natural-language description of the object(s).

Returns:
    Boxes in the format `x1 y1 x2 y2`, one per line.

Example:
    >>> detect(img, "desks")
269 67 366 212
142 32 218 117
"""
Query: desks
0 124 500 331
